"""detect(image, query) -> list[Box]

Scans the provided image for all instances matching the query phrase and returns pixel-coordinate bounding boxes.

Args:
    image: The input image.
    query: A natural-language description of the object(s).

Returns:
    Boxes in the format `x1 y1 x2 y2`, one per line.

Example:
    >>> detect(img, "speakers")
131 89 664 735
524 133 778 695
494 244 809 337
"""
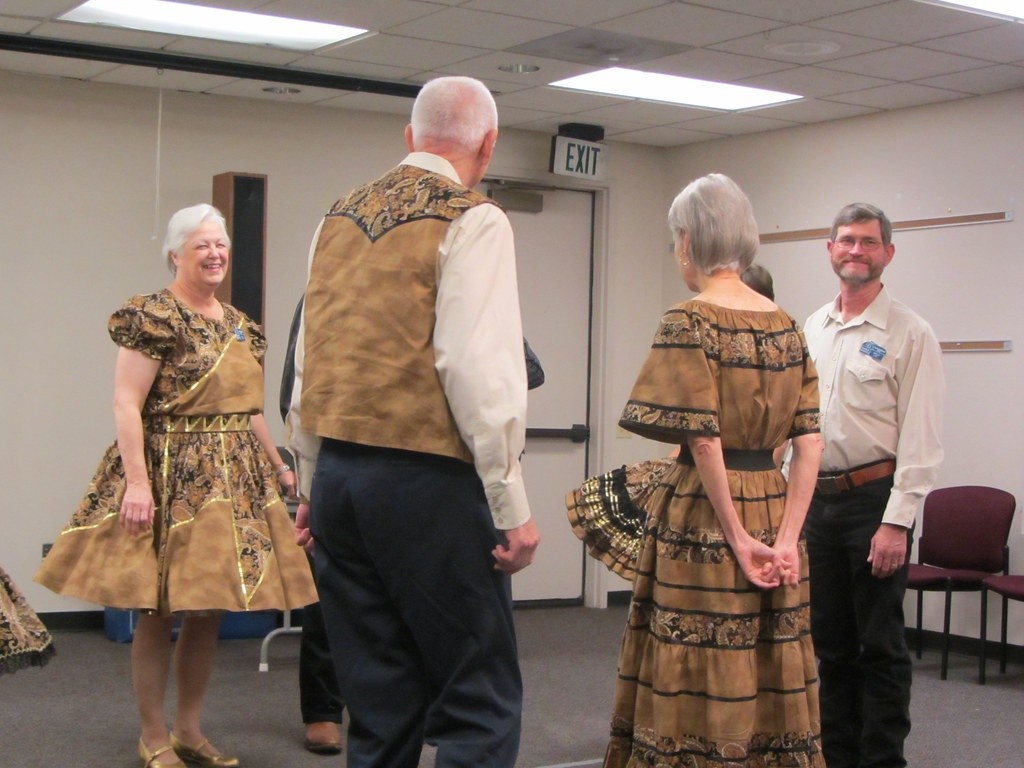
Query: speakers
212 171 267 343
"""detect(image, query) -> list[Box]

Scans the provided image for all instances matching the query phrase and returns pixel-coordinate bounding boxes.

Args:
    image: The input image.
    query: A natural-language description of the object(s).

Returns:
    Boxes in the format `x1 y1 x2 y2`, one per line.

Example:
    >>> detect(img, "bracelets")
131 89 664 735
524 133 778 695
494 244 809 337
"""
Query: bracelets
276 464 290 477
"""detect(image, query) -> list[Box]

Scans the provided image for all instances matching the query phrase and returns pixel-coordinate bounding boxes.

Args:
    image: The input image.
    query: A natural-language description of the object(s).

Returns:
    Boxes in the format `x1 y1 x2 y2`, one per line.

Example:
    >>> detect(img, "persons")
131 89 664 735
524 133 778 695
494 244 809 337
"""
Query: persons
32 204 322 768
781 201 945 768
598 172 827 768
662 263 788 478
285 75 538 768
278 294 348 755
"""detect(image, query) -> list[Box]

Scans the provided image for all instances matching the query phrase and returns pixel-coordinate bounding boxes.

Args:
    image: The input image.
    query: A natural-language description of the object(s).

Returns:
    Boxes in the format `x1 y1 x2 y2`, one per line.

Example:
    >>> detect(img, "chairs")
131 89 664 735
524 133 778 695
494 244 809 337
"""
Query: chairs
980 575 1024 685
902 485 1016 686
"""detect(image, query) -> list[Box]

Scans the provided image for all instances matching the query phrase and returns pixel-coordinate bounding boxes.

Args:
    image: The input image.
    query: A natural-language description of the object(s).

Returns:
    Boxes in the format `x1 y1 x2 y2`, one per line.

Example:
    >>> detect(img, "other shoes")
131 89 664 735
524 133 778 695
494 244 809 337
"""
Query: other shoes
170 732 243 768
138 739 188 768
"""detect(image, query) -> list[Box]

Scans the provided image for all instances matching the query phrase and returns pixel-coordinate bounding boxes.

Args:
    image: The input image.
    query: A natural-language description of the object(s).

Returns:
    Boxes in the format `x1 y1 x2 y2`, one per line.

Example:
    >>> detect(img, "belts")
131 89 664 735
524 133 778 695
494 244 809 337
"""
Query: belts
815 461 898 493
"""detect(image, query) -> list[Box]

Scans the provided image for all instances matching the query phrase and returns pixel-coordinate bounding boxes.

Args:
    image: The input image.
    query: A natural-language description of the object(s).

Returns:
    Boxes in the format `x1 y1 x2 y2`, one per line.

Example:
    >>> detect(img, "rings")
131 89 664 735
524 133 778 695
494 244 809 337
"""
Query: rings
891 563 898 569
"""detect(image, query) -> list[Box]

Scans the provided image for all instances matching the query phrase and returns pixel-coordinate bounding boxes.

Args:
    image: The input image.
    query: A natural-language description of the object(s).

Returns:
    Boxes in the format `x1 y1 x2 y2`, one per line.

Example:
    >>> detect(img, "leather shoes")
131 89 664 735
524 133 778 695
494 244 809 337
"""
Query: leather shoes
305 721 343 753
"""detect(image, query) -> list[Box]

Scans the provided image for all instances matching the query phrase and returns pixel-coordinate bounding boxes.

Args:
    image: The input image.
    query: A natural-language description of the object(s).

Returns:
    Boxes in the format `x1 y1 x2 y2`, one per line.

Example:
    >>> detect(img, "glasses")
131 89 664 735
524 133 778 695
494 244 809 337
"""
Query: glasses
831 237 885 251
